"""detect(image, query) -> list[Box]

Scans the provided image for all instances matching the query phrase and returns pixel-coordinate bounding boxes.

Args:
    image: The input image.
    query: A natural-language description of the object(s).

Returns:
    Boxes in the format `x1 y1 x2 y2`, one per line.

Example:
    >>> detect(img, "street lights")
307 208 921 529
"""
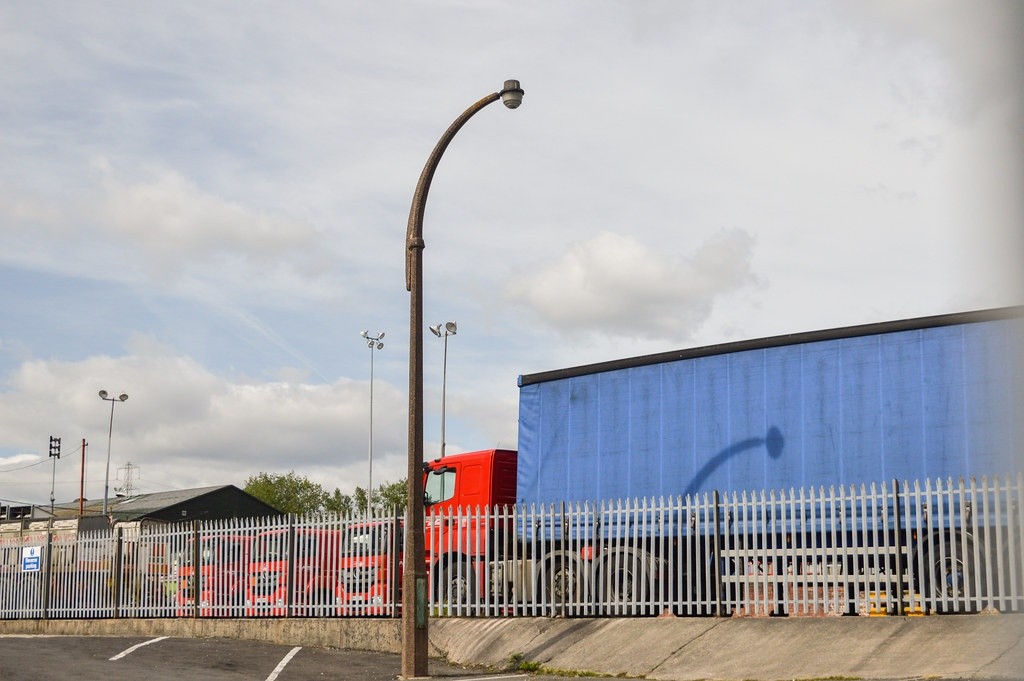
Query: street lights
99 389 129 516
430 320 457 457
361 329 387 519
393 78 526 681
46 436 61 514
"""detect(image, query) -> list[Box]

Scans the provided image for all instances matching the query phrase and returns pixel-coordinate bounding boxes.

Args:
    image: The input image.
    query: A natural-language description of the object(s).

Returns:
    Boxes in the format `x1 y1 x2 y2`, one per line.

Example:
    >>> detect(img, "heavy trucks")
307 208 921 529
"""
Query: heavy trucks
178 534 251 620
247 528 342 619
335 520 403 619
422 303 1024 615
